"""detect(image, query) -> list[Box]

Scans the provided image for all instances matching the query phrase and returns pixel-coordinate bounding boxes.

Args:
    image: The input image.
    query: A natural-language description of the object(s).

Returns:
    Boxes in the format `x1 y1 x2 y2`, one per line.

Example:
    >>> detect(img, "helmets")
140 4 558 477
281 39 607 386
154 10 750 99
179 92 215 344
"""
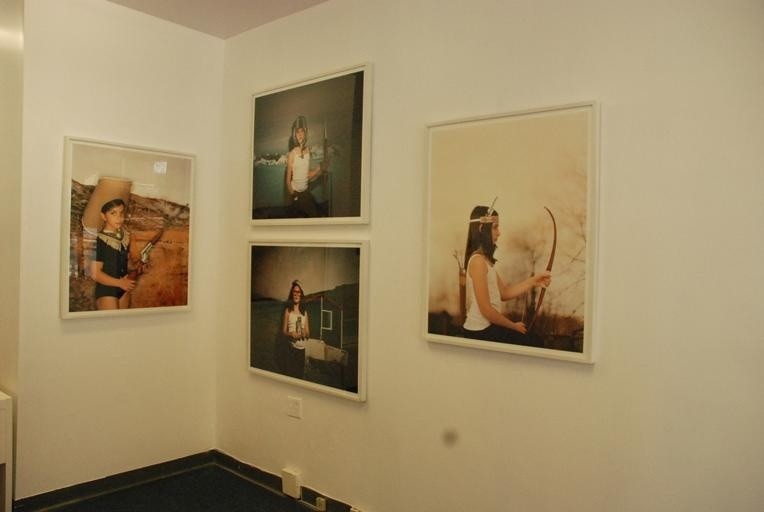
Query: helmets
291 115 308 146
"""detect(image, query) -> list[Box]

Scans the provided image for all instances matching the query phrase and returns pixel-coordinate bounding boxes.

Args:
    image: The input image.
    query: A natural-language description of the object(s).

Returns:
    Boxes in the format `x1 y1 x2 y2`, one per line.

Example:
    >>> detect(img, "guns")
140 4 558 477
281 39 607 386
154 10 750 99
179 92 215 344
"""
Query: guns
127 205 188 281
321 127 329 193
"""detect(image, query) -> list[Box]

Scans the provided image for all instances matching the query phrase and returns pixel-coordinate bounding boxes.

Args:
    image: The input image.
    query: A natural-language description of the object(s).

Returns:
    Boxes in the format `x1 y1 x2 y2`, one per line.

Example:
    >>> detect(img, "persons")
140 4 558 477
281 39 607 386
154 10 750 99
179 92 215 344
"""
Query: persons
90 198 150 310
286 116 329 218
462 206 552 342
281 280 310 373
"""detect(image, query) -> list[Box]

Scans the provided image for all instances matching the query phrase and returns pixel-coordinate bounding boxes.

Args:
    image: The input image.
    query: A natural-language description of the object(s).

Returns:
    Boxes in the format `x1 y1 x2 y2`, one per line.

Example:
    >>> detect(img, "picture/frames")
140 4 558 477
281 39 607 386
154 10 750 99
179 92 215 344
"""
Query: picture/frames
248 60 371 228
248 238 371 403
58 133 199 324
421 98 600 368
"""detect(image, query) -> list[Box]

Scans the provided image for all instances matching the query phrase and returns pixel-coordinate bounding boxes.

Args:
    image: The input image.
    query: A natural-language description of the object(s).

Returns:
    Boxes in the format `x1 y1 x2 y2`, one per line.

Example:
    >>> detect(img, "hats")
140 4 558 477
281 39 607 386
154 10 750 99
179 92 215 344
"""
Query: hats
81 175 133 237
292 279 304 296
